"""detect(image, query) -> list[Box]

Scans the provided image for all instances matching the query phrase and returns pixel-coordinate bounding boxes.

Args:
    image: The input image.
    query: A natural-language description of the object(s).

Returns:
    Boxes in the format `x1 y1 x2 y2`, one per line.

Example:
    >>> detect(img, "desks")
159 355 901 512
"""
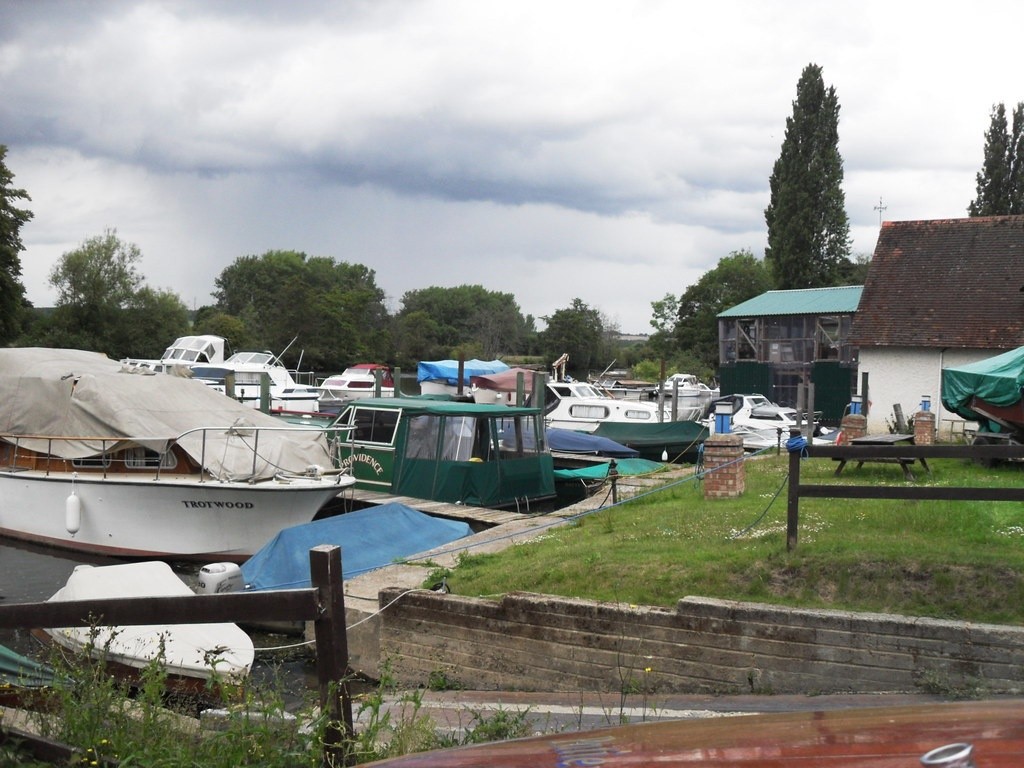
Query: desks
832 434 930 482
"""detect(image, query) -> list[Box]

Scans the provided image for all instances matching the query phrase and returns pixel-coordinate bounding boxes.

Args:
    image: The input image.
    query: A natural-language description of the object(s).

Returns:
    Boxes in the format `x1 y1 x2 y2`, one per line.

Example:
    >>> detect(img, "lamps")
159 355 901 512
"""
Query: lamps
851 394 863 402
922 395 931 401
715 402 733 414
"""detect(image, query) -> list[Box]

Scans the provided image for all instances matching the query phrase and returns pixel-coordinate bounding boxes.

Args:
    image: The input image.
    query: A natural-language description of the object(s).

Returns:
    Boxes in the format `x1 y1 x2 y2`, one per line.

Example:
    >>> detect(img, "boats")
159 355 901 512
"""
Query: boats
27 559 257 708
1 333 1024 768
